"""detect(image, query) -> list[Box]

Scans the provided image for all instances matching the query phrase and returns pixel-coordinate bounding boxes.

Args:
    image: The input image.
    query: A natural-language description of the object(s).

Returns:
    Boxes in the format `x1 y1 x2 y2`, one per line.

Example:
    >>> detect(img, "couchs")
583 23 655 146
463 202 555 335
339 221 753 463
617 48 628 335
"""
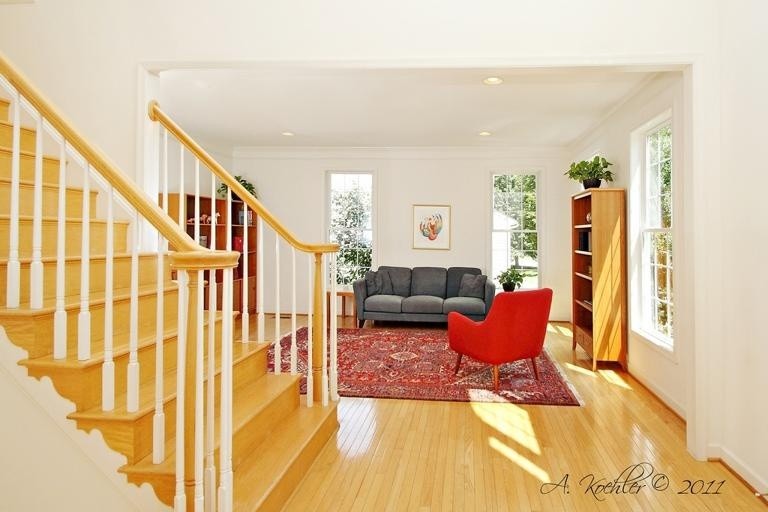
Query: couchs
352 266 495 324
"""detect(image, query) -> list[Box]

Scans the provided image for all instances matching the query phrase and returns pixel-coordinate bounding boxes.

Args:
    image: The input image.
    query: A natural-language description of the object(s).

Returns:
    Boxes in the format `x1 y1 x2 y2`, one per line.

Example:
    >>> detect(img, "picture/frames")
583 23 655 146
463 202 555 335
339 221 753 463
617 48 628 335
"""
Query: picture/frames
412 204 452 251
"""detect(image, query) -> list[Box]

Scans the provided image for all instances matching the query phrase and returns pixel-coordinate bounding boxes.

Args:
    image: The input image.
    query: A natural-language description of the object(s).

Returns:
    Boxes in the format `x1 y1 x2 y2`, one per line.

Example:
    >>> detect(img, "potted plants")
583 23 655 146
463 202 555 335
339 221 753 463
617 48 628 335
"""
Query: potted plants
218 175 257 200
564 156 615 190
496 269 524 292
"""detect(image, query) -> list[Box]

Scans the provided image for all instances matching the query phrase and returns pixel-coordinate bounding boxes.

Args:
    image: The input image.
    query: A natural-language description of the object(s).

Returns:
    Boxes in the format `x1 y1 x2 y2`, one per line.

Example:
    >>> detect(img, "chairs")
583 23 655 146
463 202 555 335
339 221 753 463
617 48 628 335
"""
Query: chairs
448 288 552 391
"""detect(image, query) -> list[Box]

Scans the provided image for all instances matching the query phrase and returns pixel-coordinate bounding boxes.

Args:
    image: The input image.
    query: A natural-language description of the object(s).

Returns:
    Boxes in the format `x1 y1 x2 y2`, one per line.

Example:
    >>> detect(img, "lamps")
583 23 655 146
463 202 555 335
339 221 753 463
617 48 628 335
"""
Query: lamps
482 76 505 87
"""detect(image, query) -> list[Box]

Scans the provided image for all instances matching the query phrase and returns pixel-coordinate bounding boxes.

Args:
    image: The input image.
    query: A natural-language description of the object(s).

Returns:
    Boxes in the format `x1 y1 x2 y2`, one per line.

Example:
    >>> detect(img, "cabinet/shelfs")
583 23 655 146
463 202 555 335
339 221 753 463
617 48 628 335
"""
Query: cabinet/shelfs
569 188 627 371
159 191 258 318
